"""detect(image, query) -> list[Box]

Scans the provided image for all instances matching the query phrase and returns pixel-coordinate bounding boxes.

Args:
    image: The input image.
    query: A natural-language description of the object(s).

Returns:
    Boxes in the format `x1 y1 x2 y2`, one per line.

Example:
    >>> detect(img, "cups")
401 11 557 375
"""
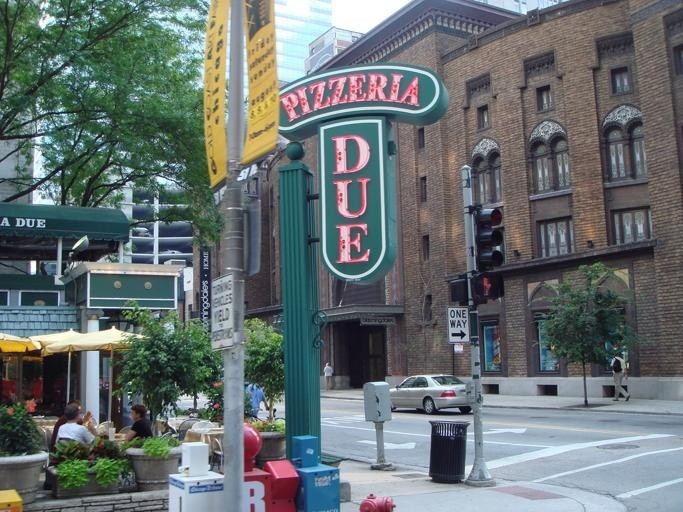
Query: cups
108 427 116 442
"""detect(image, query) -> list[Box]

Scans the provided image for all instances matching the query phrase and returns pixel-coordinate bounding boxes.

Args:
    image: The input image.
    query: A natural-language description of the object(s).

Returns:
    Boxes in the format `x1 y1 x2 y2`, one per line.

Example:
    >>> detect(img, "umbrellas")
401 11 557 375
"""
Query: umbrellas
1 326 149 421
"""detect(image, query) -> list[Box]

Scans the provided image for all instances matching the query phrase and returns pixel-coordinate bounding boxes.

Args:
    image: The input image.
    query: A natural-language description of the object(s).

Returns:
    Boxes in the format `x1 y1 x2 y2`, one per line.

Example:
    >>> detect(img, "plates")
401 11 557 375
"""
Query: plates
32 415 44 418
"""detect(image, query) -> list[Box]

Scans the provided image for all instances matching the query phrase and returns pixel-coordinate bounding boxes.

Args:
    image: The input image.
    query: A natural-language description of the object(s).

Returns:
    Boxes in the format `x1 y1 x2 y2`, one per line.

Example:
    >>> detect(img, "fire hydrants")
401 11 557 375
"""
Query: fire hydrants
358 494 395 512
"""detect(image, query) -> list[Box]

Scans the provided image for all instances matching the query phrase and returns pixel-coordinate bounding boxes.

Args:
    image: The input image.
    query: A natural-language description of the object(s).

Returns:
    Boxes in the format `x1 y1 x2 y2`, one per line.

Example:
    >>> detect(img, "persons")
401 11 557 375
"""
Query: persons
50 400 98 454
610 353 630 402
323 363 334 391
125 405 153 441
243 380 270 419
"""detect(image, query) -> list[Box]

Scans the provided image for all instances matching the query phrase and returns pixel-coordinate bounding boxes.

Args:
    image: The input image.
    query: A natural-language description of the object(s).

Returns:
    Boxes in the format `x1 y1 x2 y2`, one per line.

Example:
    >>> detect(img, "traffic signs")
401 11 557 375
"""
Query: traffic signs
448 307 470 343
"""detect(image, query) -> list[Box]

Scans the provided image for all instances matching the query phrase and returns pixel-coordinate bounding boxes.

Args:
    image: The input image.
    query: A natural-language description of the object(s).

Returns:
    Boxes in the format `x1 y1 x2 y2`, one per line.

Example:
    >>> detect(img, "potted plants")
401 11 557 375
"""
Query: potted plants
45 457 120 499
245 320 286 468
121 433 182 490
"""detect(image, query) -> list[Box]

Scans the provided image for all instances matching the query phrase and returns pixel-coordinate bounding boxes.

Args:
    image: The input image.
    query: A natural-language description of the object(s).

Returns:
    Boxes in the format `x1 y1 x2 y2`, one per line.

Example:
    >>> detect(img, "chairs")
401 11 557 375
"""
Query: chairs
30 415 224 474
0 379 44 415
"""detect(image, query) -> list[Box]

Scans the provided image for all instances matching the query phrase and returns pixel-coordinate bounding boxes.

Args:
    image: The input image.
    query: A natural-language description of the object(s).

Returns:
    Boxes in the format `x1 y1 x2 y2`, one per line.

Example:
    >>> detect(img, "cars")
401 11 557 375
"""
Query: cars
389 374 471 415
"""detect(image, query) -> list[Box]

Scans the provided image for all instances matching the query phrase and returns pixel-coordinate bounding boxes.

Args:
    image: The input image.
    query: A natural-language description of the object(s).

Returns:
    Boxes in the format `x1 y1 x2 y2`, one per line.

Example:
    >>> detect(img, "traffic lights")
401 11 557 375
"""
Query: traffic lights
473 206 504 269
470 271 505 303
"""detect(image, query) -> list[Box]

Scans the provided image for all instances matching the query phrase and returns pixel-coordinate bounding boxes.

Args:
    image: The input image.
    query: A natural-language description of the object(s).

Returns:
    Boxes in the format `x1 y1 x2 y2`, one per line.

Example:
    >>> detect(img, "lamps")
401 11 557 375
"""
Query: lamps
69 235 89 261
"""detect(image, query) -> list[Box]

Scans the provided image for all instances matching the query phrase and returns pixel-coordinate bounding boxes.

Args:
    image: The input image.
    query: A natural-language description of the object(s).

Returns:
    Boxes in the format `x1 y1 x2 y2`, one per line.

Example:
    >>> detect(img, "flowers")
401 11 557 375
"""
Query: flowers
0 399 44 454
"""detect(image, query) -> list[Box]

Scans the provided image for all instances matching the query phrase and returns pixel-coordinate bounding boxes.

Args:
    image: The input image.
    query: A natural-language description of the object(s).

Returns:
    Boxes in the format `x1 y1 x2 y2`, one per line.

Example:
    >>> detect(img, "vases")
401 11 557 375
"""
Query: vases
0 451 48 504
